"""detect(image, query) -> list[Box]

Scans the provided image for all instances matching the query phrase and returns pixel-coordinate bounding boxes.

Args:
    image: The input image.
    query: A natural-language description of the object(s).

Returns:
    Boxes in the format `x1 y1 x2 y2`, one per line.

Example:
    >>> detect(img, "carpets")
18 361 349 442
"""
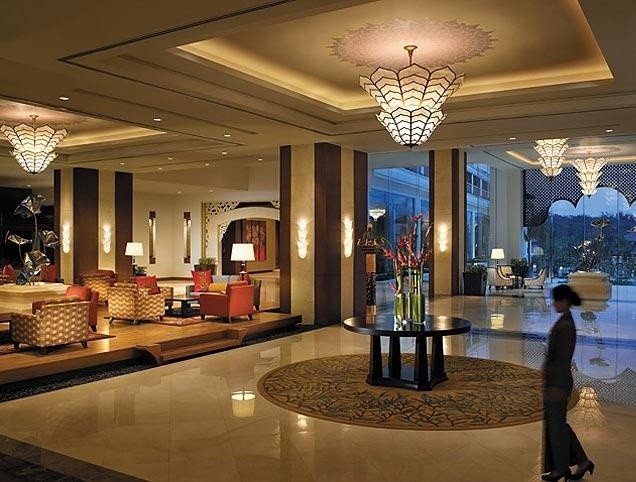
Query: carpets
257 351 581 432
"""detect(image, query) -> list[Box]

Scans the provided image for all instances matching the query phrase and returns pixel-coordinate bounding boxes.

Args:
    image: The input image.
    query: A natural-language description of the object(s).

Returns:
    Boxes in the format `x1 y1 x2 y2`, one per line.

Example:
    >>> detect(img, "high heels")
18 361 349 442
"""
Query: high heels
541 468 572 482
571 459 595 480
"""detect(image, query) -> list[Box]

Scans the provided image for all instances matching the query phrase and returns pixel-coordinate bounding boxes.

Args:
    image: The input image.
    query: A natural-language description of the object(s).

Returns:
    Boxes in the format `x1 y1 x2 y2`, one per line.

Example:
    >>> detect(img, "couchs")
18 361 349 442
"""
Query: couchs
106 281 166 325
487 267 512 290
497 265 522 289
134 275 174 310
10 296 92 355
81 273 110 306
523 269 545 289
185 275 261 311
199 280 254 323
64 286 99 331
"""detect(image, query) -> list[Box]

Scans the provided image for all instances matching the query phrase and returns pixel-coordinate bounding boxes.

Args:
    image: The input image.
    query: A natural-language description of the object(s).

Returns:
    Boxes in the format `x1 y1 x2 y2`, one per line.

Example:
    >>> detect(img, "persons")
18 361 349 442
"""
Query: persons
540 284 595 482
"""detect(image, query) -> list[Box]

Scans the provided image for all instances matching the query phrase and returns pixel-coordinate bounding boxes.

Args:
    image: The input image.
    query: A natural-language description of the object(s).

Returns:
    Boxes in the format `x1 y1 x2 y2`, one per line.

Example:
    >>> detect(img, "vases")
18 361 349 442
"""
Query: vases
411 269 422 325
393 269 405 324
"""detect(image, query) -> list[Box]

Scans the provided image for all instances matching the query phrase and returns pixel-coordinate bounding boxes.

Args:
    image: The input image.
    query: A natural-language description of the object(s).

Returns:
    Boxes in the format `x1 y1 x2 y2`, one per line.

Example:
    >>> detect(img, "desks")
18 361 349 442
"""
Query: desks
343 313 471 391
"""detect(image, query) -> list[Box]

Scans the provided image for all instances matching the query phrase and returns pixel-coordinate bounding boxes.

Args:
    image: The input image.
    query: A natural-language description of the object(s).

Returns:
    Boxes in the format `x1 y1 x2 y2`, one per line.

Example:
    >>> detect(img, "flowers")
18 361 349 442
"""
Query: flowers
376 226 413 269
394 214 434 269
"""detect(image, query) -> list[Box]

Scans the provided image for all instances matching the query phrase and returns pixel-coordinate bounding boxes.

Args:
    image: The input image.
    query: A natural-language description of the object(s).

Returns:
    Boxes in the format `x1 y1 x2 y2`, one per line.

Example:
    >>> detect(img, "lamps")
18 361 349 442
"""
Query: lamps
0 122 71 175
62 223 70 254
124 242 144 256
296 219 308 260
359 64 465 149
437 223 448 252
230 243 255 262
102 224 111 254
532 139 569 181
570 156 608 199
490 248 504 260
231 391 255 418
344 220 353 258
369 209 385 222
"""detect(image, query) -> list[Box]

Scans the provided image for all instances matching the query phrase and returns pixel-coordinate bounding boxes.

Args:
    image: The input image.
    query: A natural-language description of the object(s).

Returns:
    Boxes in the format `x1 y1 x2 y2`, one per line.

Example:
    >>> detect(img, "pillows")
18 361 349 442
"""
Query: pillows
134 275 159 294
191 269 212 292
